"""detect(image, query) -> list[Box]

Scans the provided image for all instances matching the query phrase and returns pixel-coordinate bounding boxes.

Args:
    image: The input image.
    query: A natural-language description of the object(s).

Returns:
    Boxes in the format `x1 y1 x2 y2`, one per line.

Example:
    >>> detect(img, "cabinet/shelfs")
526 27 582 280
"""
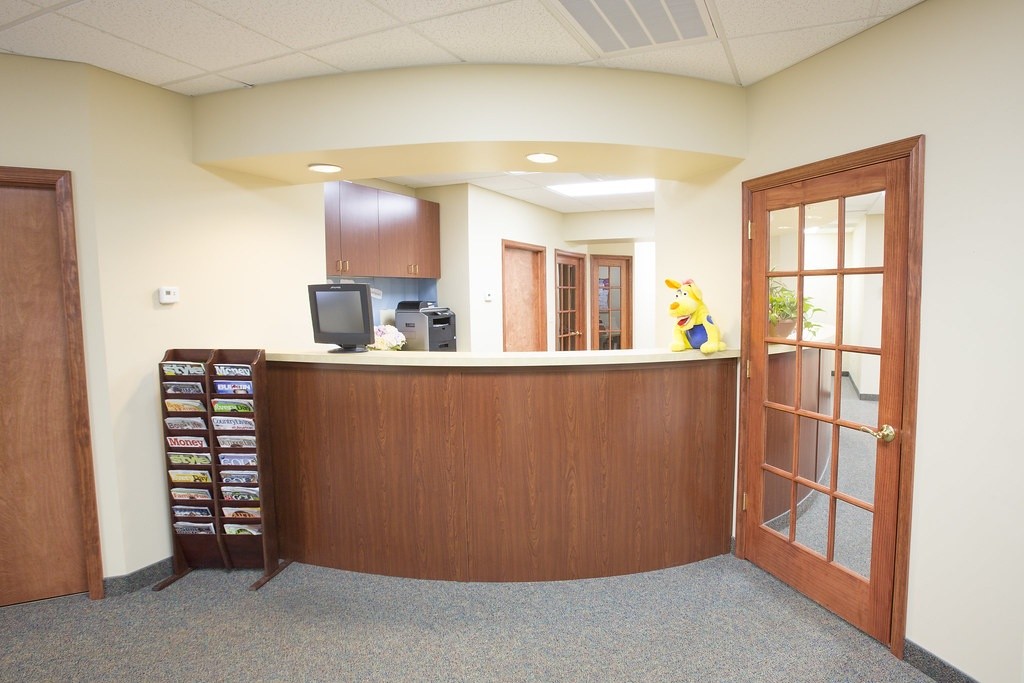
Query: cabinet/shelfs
324 180 379 276
377 188 439 278
150 348 295 591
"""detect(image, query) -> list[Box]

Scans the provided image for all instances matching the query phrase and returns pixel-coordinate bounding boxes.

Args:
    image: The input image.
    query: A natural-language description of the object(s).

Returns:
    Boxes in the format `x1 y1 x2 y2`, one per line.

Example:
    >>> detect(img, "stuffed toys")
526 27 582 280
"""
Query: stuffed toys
664 276 727 354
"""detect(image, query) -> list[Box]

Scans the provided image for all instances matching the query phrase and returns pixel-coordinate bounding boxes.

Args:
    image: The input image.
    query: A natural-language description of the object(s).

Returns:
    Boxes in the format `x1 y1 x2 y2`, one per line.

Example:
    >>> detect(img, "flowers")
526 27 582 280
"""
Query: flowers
366 323 407 350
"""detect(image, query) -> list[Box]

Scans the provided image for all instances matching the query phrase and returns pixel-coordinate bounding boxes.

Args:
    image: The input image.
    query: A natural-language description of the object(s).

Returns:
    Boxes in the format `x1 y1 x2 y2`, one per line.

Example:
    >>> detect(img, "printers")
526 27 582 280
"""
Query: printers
395 301 457 352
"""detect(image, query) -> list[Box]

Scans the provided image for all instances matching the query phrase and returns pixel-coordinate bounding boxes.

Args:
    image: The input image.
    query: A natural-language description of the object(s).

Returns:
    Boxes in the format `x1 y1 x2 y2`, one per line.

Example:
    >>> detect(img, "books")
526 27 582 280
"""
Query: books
160 360 262 536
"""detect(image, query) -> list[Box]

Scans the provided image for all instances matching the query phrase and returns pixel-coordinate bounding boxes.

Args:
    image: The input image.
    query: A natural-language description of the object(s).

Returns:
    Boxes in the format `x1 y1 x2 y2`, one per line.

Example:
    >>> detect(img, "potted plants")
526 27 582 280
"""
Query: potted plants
767 265 826 344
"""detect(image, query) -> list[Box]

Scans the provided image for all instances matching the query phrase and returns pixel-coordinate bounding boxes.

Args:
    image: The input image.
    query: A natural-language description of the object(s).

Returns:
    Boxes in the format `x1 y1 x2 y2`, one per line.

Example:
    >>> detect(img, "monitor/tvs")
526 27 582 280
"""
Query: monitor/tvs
308 283 375 354
